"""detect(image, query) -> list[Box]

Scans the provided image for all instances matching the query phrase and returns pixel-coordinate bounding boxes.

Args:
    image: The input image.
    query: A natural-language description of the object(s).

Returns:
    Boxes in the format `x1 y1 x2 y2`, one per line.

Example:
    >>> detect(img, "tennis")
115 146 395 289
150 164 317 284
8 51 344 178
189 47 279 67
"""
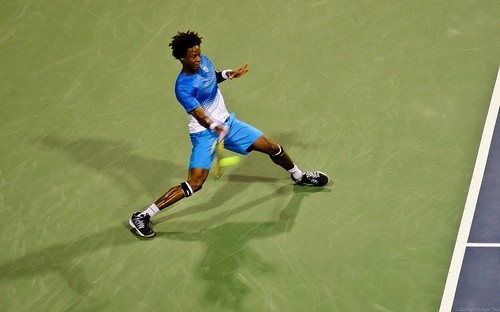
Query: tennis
219 156 240 165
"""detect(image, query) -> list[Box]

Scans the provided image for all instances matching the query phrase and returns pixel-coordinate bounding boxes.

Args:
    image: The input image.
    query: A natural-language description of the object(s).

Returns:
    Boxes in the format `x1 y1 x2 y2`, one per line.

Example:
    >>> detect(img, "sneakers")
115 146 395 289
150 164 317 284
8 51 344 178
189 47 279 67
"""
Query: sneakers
128 212 156 237
291 171 331 187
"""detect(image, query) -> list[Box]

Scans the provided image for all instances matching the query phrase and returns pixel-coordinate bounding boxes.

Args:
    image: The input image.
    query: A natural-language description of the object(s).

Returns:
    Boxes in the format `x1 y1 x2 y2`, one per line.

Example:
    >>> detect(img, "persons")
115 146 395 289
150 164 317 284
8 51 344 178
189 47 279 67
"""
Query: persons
129 29 330 238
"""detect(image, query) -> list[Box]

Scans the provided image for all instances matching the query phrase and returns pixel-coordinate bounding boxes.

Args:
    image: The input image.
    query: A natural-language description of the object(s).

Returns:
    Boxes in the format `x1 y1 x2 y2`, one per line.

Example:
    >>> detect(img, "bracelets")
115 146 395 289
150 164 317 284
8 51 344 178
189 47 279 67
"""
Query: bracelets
210 123 219 135
222 69 232 80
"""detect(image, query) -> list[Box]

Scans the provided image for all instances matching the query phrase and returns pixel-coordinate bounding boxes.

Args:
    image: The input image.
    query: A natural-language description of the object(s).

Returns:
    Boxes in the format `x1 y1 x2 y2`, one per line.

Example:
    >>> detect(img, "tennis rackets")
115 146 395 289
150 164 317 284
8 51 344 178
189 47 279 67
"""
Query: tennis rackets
210 129 225 180
